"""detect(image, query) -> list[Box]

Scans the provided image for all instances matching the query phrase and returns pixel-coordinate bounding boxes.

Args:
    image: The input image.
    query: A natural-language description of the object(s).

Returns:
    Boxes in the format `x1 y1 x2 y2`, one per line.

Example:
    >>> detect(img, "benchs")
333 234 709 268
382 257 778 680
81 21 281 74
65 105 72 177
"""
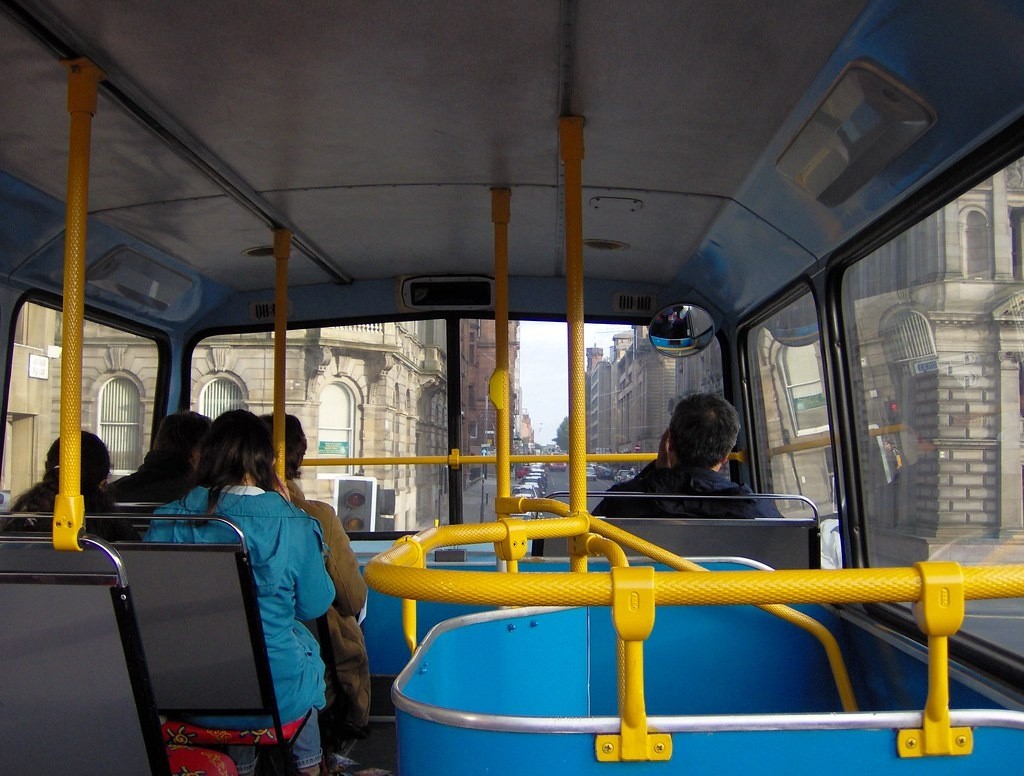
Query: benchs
0 502 344 776
530 491 821 571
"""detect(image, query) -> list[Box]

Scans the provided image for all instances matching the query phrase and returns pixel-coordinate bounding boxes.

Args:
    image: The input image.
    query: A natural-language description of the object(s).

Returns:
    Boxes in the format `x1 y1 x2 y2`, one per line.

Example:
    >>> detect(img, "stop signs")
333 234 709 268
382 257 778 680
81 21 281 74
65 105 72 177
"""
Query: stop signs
635 445 640 452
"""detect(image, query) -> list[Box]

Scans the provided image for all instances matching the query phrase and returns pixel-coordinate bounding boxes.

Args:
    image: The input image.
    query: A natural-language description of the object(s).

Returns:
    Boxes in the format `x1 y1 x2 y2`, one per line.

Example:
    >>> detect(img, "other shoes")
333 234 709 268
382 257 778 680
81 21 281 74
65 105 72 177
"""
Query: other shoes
295 752 337 776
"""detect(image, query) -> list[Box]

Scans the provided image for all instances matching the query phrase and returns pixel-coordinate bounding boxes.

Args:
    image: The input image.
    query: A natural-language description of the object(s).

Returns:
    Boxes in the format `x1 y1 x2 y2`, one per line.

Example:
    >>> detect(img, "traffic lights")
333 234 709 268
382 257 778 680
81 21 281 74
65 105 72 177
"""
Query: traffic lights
375 485 395 532
884 400 901 424
334 475 377 532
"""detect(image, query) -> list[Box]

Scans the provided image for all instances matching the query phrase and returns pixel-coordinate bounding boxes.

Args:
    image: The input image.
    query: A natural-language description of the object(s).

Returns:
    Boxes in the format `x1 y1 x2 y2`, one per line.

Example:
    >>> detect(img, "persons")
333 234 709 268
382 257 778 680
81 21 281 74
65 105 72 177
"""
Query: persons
660 305 684 324
108 411 213 504
589 393 784 519
876 371 971 533
0 430 142 542
142 410 335 776
259 413 368 740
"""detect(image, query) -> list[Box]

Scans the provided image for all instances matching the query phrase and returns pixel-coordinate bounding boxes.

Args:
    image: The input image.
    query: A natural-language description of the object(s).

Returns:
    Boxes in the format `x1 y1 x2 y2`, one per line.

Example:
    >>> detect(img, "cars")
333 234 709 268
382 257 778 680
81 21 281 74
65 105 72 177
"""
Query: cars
586 467 596 481
614 470 636 484
510 464 548 520
589 464 613 480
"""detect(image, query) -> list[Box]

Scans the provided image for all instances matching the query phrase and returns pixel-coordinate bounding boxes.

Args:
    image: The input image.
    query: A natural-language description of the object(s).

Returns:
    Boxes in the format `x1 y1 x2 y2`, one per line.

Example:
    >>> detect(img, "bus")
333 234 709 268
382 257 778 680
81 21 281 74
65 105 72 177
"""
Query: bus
548 452 566 472
